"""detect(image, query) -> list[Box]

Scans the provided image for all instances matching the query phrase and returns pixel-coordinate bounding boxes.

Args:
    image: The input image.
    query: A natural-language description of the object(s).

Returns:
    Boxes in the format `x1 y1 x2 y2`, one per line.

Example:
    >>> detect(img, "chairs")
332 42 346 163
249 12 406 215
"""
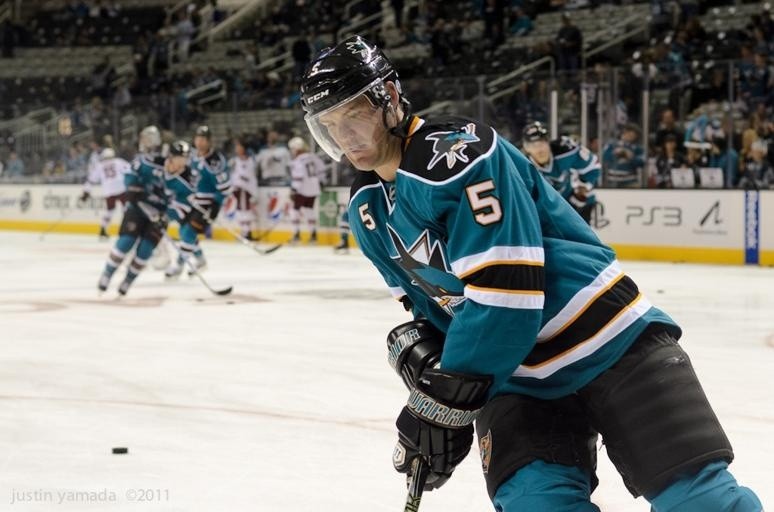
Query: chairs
1 2 774 196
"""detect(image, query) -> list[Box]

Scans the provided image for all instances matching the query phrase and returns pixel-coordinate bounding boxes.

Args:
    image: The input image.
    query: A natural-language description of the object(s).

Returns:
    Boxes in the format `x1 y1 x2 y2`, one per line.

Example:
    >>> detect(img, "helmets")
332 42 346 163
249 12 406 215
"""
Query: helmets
139 124 212 158
522 120 550 154
298 35 403 163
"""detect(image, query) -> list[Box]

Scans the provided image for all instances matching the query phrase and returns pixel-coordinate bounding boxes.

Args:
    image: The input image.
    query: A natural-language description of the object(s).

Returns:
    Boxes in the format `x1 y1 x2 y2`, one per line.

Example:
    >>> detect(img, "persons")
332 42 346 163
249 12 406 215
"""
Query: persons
299 36 765 512
0 0 774 295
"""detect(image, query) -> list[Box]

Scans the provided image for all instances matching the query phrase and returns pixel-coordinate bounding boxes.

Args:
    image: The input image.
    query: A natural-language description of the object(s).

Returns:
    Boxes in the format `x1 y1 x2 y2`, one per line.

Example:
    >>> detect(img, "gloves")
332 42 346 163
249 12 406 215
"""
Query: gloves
386 318 446 389
393 368 495 492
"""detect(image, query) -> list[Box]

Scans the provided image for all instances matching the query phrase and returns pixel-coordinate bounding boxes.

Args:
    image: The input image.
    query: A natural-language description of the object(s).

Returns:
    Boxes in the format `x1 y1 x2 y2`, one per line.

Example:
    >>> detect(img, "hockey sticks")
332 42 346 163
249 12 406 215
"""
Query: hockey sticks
159 217 231 295
214 219 281 255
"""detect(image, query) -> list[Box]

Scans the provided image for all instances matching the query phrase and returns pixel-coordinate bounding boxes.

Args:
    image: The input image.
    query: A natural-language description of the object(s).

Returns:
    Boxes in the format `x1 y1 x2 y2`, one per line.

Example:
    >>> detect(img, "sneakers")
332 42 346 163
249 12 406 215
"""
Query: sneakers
99 274 110 290
166 265 182 277
188 258 206 275
118 280 130 295
